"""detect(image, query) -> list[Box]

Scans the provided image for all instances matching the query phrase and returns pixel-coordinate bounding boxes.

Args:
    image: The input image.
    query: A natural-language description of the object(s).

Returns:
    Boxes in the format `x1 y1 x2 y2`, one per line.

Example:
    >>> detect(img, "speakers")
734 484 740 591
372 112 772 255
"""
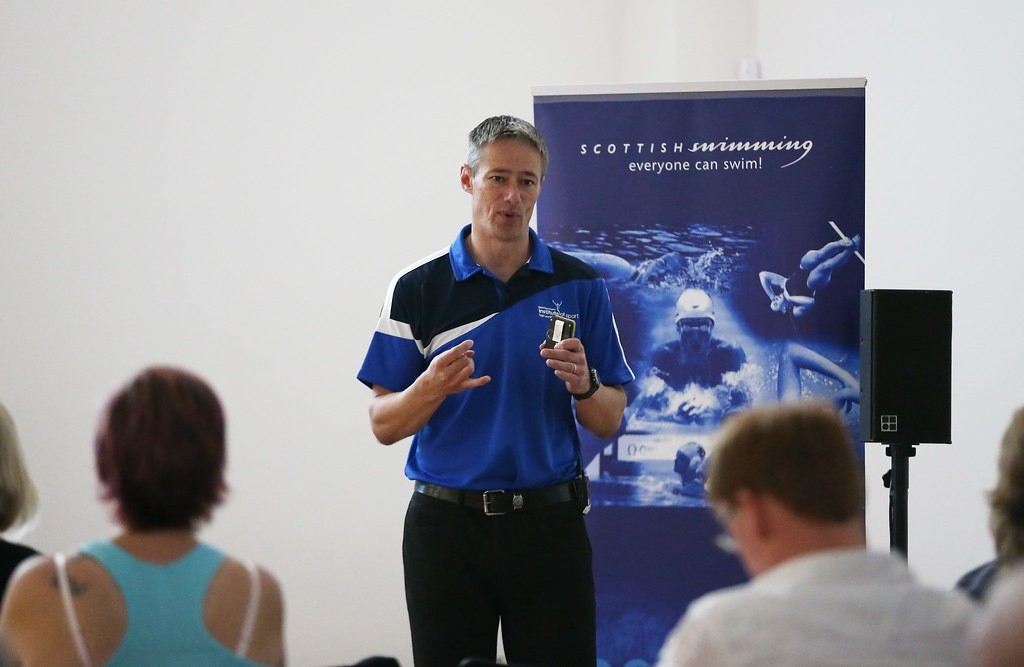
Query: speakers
858 289 951 446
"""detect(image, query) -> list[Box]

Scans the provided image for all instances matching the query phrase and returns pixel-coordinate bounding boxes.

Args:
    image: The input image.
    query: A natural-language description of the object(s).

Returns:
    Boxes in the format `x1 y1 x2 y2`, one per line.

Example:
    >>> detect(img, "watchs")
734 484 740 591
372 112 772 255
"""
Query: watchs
569 368 600 401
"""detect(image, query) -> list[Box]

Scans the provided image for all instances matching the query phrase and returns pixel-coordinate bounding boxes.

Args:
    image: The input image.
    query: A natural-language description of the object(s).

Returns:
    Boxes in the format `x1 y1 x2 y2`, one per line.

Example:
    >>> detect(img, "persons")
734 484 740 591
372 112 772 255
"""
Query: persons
648 289 752 425
356 115 637 667
0 365 289 667
672 342 862 503
561 245 686 286
657 405 1024 667
759 235 861 316
0 403 46 614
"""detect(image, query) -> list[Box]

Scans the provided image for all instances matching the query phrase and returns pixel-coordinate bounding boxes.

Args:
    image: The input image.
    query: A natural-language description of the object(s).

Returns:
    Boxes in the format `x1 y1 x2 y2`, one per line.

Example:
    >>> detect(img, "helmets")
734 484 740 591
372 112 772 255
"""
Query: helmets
675 289 714 327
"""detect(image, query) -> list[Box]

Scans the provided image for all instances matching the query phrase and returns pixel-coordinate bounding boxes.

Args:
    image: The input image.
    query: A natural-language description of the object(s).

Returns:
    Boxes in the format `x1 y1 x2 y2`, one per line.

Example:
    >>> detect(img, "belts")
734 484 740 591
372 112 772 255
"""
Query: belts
415 474 589 516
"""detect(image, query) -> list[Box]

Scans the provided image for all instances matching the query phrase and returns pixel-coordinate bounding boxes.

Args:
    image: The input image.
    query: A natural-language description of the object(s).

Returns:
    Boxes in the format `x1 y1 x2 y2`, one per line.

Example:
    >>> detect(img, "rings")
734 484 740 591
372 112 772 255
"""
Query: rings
572 362 576 373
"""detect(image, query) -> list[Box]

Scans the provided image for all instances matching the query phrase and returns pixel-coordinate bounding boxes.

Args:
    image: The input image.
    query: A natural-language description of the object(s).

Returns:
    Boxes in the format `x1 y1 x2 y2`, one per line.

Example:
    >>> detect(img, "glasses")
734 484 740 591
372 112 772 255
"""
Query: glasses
712 510 741 555
678 321 711 335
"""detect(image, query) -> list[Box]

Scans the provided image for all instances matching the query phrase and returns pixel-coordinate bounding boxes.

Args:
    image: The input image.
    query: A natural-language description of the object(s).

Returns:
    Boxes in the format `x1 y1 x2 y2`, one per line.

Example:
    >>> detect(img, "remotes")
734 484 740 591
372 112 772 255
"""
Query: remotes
546 316 576 350
